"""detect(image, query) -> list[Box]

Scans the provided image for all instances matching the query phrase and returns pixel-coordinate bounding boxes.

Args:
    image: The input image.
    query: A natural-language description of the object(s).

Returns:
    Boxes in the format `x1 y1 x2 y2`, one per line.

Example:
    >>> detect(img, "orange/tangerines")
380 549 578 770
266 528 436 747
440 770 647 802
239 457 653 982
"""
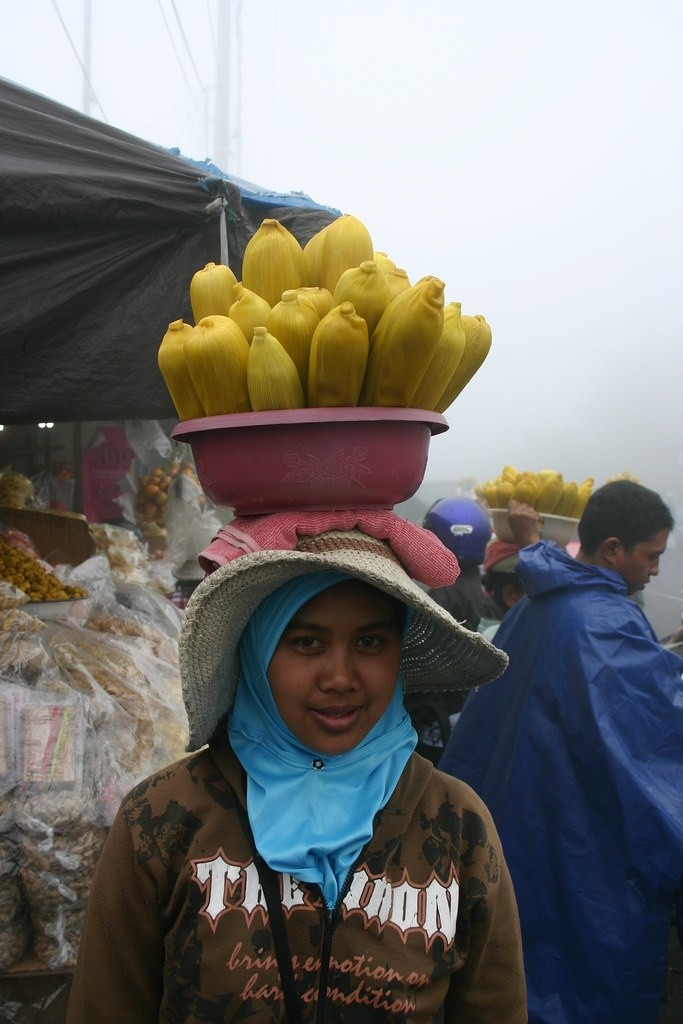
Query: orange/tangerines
133 463 209 532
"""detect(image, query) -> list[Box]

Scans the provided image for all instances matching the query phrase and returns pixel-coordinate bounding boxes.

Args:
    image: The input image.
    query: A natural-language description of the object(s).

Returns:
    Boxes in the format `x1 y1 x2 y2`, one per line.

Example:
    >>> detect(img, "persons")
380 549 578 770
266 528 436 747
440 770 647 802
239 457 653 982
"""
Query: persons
478 497 541 652
411 492 501 764
65 505 529 1024
437 479 683 1024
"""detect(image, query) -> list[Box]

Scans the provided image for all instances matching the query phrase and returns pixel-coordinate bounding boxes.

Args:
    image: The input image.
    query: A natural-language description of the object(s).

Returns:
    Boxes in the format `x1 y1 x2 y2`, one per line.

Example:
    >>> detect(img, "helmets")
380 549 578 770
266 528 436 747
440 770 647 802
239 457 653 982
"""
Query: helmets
425 496 492 563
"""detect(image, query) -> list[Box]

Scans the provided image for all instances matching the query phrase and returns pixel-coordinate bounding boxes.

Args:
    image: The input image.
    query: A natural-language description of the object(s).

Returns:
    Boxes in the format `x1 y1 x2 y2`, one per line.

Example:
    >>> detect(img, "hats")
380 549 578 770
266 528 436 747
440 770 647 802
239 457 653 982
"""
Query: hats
179 525 508 752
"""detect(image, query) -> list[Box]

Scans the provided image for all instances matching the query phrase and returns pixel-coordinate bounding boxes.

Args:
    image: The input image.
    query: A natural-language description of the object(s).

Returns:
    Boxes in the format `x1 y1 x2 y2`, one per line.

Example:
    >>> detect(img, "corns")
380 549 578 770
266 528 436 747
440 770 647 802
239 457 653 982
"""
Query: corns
157 214 494 421
479 464 594 522
